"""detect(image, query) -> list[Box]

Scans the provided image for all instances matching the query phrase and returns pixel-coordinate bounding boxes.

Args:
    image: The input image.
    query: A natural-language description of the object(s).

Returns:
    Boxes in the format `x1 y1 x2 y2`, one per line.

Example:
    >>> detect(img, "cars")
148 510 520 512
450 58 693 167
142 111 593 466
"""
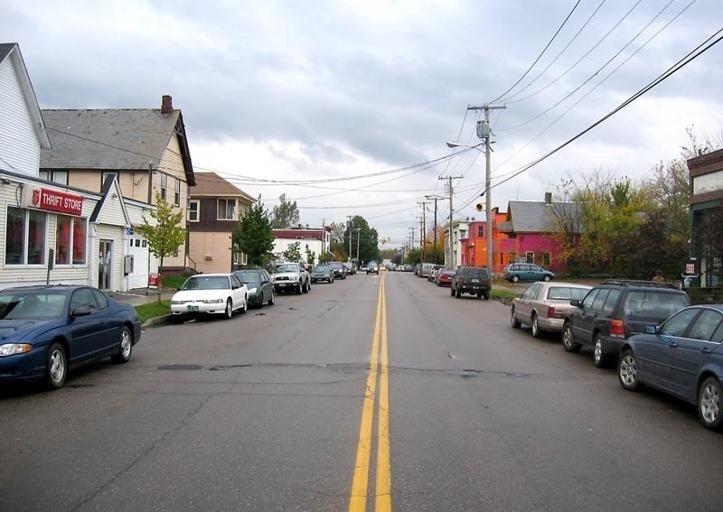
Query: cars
0 282 144 393
615 301 723 432
231 267 276 311
412 260 457 287
273 258 357 296
359 260 414 275
508 280 595 338
168 272 251 322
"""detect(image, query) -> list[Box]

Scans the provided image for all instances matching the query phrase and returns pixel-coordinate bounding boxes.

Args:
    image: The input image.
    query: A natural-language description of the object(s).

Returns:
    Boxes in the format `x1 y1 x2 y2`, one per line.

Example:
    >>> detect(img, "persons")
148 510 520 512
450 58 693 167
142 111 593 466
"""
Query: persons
652 269 664 282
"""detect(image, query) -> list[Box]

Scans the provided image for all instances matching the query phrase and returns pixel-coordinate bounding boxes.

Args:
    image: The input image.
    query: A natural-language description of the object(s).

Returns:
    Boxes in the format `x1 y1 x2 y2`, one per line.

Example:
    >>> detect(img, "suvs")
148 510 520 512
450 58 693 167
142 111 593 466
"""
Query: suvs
449 264 492 301
559 277 695 370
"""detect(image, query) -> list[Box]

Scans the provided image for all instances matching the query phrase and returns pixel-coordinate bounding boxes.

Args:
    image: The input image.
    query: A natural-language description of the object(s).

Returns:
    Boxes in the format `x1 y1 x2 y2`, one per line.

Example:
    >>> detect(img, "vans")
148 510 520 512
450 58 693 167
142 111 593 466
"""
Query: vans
503 262 556 283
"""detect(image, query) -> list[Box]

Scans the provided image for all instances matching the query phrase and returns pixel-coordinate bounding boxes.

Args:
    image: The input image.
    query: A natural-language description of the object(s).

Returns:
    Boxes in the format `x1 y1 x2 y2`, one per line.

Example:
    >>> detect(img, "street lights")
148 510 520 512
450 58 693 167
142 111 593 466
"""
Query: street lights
444 140 495 287
424 194 453 267
